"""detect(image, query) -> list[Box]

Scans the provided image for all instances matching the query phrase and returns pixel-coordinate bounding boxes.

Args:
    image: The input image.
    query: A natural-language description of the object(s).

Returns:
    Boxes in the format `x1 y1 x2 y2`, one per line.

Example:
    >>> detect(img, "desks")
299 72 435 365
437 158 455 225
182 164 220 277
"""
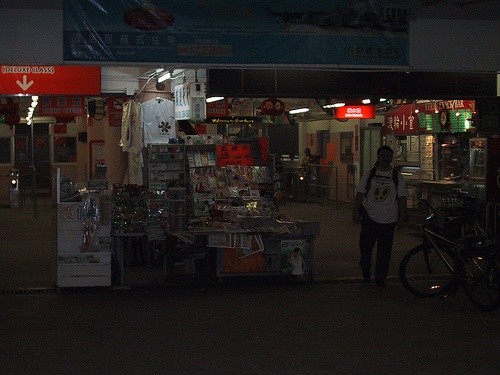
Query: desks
405 178 463 211
110 230 313 277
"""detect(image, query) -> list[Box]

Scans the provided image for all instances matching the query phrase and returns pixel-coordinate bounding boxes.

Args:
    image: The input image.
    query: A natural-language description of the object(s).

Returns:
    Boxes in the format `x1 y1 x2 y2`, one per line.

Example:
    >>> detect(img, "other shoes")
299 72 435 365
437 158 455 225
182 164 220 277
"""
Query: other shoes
364 272 370 279
376 279 385 287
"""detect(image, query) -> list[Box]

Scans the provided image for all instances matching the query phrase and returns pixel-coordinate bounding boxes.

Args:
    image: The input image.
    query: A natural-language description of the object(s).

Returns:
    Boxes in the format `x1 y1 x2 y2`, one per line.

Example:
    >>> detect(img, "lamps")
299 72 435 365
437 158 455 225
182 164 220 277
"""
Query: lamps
260 97 285 122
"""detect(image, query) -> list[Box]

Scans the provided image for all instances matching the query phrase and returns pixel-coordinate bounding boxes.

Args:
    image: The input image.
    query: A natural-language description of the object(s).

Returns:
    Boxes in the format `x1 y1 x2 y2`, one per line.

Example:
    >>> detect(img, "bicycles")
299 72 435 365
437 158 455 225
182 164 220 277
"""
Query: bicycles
398 190 500 312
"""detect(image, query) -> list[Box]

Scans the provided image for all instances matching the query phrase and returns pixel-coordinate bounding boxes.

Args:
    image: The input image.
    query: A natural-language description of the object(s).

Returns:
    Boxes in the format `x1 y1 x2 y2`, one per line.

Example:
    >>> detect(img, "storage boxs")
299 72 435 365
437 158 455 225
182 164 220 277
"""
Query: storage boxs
297 220 321 235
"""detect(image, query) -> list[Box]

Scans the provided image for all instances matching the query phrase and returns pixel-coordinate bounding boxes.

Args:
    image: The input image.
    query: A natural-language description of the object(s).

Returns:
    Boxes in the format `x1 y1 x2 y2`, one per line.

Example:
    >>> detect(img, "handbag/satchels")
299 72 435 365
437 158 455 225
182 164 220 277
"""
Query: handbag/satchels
352 201 363 224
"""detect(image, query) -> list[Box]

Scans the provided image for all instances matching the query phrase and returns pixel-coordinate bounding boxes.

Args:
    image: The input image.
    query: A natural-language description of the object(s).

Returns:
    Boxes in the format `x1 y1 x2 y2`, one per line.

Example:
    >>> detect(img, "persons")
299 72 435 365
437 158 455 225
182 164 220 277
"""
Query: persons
353 145 407 283
298 148 320 172
288 247 305 275
